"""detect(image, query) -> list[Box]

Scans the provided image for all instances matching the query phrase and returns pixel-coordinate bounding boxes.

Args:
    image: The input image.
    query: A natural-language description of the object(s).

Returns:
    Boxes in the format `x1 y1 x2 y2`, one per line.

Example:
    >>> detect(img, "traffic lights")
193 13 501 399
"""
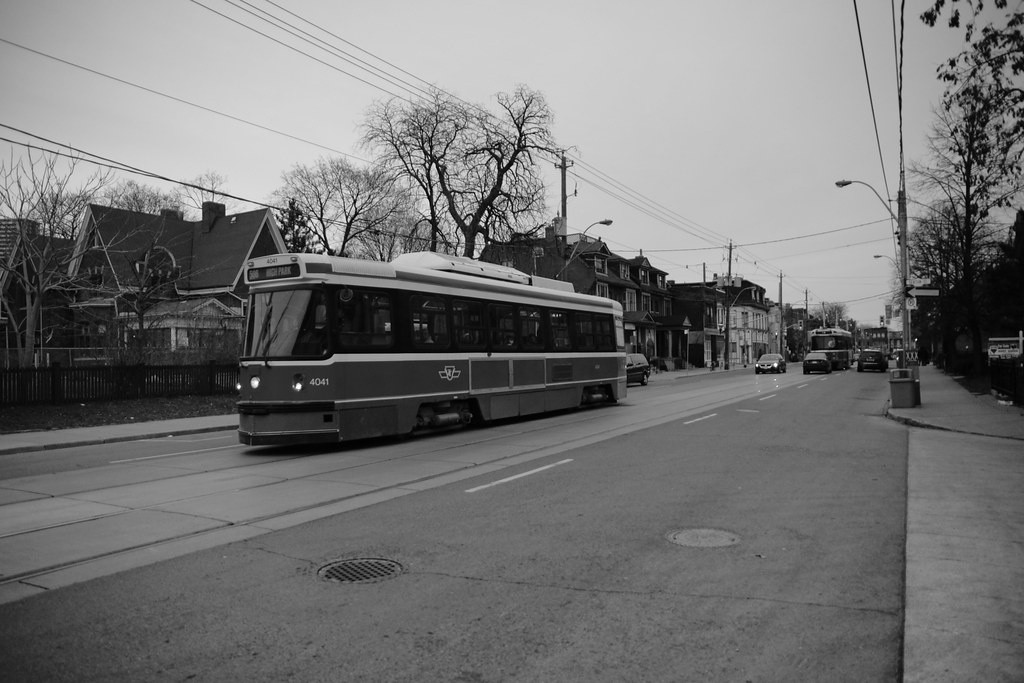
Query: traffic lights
880 316 885 327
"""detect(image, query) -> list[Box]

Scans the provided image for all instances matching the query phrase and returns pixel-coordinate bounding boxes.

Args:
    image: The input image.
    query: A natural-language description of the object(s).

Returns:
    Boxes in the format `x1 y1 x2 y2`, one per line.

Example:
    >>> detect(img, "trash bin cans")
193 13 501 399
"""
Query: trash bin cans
888 368 916 408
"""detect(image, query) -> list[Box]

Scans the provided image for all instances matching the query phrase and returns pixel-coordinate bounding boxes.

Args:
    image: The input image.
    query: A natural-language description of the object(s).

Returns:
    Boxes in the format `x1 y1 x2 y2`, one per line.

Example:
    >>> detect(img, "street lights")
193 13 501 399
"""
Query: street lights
724 286 756 370
779 299 808 354
872 255 902 275
835 180 907 376
560 219 613 281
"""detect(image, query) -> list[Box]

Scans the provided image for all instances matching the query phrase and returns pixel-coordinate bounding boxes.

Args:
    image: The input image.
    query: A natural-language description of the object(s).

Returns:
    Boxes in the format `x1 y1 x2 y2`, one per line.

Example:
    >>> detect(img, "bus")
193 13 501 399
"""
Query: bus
809 326 854 369
234 250 628 448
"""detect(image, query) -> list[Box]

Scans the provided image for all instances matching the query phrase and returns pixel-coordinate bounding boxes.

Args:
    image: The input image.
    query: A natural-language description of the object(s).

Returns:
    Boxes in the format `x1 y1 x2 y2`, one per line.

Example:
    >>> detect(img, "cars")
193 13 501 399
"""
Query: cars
755 354 787 374
802 352 833 374
857 349 887 372
626 353 650 386
